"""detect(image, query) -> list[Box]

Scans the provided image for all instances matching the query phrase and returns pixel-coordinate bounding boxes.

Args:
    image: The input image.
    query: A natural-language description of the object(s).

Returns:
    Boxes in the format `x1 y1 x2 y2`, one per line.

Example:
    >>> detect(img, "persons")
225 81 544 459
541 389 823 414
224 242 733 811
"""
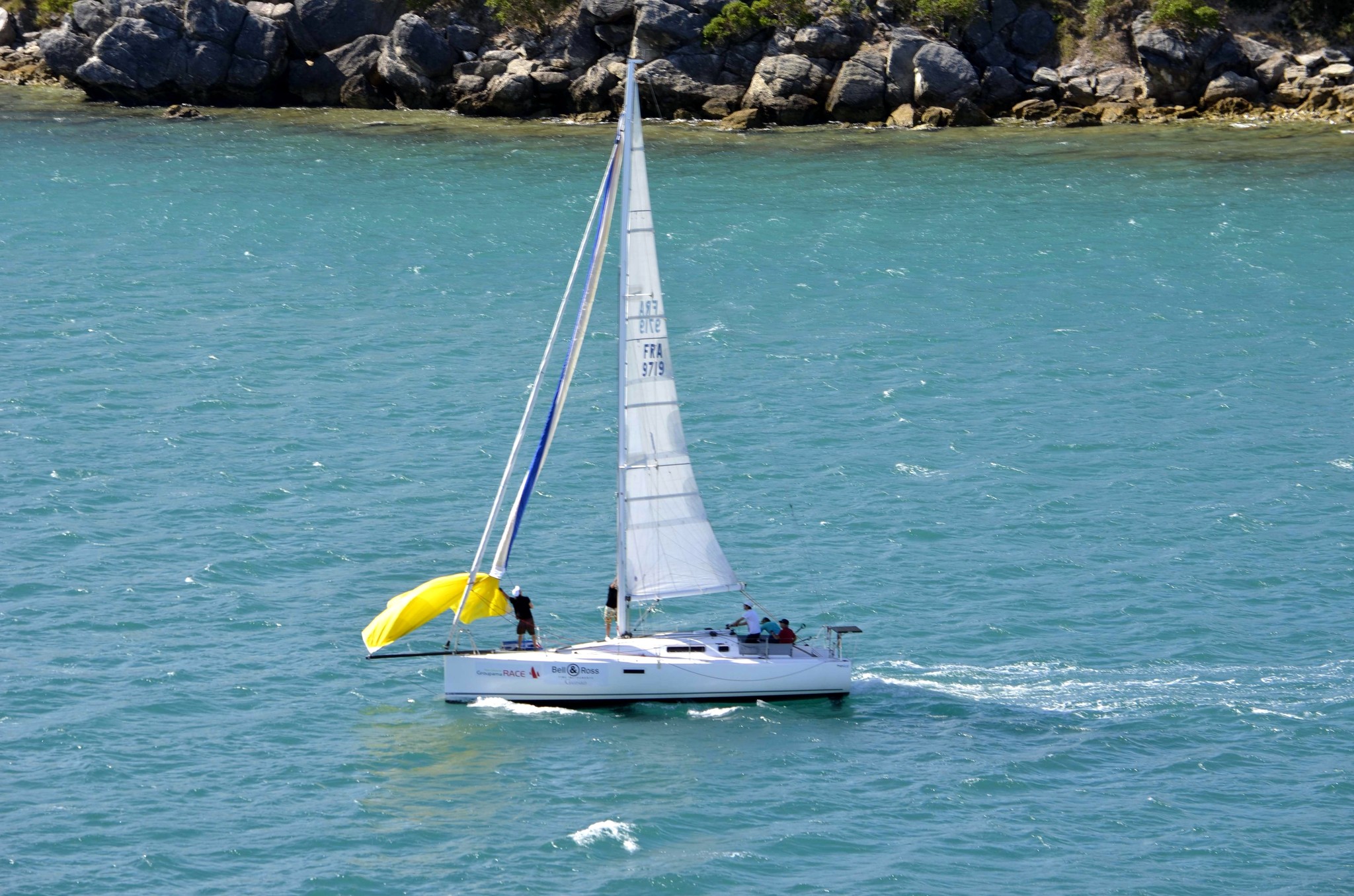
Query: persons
604 577 619 641
770 619 797 644
729 601 761 643
499 586 537 649
760 617 781 635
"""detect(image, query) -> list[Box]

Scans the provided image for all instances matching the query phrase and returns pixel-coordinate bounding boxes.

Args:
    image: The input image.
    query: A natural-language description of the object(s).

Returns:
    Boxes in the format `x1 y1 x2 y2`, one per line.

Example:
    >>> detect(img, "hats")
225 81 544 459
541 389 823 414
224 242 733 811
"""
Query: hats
743 601 753 608
778 619 789 625
512 585 520 597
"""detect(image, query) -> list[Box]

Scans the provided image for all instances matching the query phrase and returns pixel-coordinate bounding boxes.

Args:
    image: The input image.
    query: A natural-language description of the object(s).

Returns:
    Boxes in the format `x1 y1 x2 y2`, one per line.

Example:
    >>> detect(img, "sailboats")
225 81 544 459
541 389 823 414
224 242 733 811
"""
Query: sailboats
359 9 858 704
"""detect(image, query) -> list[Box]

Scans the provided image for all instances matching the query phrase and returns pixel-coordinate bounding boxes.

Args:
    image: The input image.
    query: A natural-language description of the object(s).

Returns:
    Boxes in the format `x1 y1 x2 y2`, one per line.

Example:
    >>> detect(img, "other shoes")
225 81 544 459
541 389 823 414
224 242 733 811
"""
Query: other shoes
605 636 611 642
